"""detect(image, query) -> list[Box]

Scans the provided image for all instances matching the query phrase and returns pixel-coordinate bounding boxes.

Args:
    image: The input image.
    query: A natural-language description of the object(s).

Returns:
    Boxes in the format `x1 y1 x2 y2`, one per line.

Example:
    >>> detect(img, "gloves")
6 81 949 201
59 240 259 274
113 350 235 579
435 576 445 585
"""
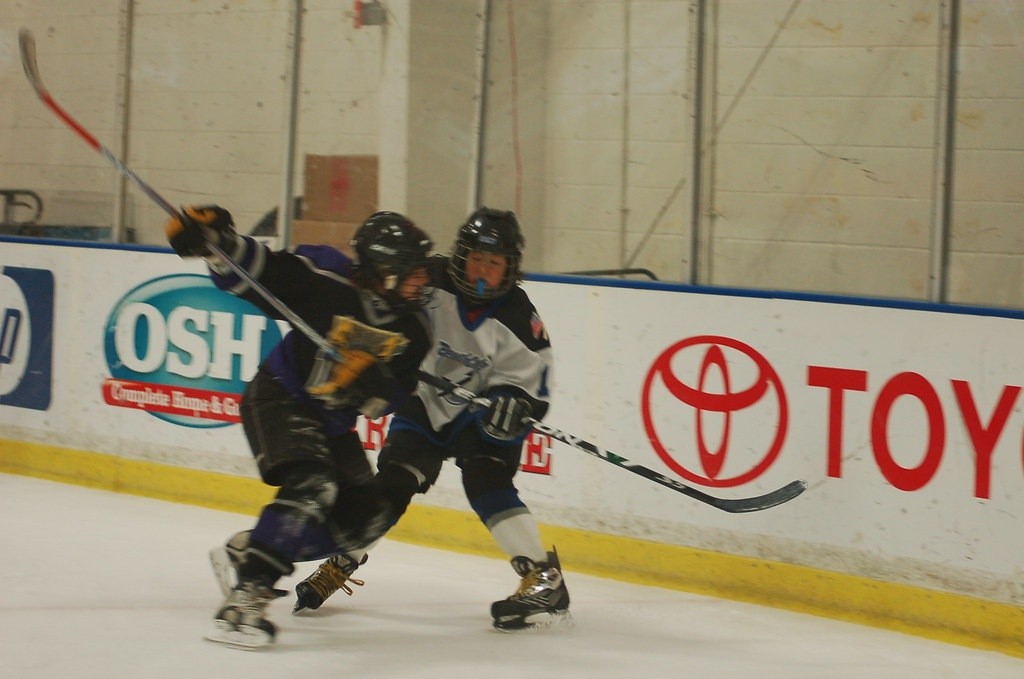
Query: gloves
165 205 237 258
306 348 394 417
482 396 532 441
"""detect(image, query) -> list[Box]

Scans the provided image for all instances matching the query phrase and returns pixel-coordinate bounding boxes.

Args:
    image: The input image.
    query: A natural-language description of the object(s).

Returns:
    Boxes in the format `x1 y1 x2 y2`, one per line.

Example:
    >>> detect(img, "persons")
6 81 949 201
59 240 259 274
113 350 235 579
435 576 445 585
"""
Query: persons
167 203 433 651
294 207 571 634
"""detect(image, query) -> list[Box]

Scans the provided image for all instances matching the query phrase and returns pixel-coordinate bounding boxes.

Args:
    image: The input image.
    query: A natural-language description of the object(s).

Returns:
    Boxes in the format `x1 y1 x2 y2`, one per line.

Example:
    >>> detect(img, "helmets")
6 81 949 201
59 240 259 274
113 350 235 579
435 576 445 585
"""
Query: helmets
447 205 526 304
350 211 435 311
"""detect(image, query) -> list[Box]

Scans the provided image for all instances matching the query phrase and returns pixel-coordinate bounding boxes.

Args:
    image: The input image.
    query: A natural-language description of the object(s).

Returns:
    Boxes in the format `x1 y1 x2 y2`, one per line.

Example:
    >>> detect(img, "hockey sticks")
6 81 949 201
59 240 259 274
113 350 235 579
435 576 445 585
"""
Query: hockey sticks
17 24 344 359
413 367 806 515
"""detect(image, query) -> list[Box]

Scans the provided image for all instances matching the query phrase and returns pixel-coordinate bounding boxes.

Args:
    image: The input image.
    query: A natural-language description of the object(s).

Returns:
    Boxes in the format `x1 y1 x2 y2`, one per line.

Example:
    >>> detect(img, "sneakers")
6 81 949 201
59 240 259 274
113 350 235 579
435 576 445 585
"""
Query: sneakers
210 529 255 597
491 544 575 634
291 551 369 615
203 574 289 651
